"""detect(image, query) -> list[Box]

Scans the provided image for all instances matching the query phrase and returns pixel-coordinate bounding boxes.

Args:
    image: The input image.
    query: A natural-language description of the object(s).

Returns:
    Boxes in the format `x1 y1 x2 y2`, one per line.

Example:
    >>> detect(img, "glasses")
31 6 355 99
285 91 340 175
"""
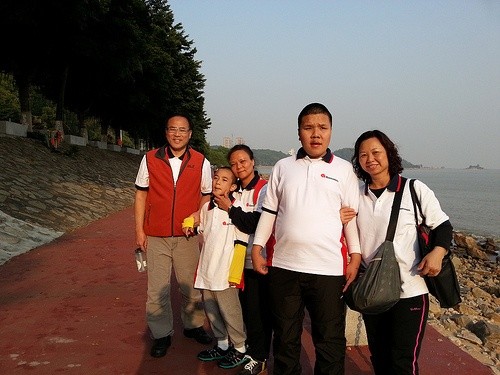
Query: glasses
166 125 192 135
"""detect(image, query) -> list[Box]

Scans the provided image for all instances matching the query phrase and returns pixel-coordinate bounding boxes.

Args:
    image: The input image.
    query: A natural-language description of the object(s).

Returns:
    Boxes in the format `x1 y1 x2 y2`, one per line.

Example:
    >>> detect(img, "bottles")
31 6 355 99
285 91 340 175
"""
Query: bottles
135 248 148 272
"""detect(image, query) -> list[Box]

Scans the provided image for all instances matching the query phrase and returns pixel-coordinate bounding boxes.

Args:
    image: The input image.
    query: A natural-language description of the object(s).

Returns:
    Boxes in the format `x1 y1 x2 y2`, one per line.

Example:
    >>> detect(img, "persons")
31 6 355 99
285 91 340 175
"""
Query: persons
107 134 114 145
182 166 250 369
116 136 122 146
340 130 454 375
134 116 212 358
250 104 360 375
214 145 277 375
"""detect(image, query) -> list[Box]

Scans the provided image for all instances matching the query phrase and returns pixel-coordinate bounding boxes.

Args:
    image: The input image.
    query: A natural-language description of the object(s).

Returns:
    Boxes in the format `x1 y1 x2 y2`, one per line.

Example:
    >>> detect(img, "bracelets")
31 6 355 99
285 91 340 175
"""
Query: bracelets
228 204 233 213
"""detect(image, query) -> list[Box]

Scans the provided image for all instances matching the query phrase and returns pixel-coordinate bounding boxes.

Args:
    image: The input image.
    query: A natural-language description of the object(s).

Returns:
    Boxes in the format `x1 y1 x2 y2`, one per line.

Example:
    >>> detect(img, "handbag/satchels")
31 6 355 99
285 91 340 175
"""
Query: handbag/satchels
409 178 463 308
345 242 402 317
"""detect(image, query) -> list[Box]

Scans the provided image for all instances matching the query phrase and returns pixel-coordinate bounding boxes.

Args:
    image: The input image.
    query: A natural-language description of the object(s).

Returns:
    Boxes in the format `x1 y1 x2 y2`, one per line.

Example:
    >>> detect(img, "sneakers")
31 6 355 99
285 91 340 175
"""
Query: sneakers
218 344 254 368
240 359 266 375
197 347 229 361
150 335 172 358
183 325 212 345
